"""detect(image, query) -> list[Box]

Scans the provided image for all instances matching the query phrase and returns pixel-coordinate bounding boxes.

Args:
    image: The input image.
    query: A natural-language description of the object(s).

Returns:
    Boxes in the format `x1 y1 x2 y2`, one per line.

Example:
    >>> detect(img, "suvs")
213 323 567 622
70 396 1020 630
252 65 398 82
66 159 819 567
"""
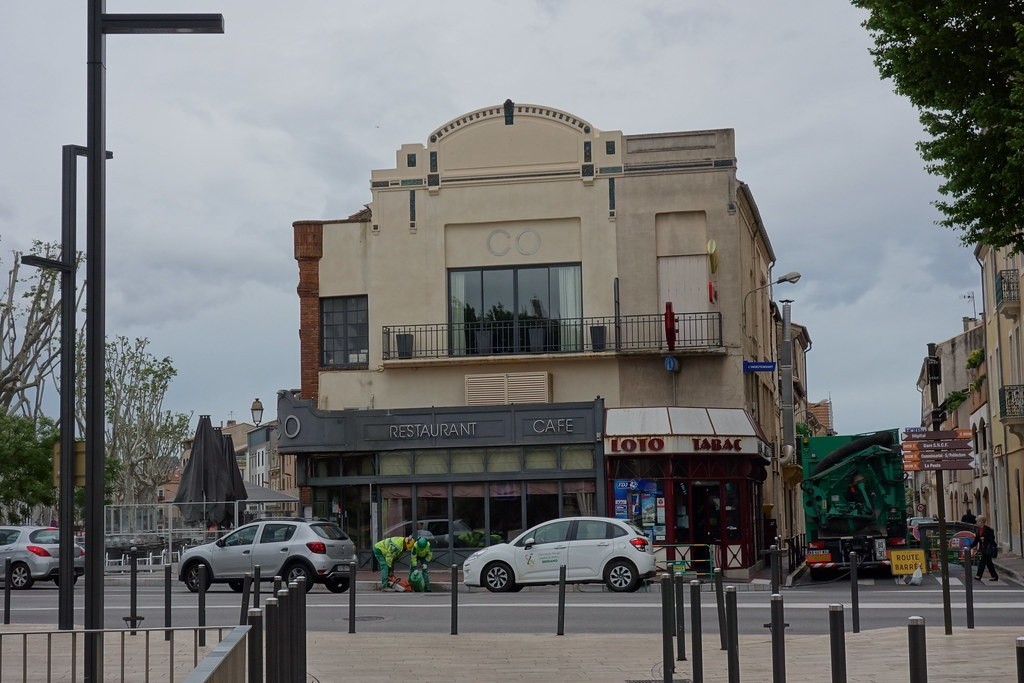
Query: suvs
380 518 472 548
178 518 358 594
0 525 85 590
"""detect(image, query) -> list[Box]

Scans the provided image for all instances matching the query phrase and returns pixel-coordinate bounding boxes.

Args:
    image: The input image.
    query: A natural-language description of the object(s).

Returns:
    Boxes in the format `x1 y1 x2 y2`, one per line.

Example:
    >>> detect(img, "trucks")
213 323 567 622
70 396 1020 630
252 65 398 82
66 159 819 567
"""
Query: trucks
801 427 910 582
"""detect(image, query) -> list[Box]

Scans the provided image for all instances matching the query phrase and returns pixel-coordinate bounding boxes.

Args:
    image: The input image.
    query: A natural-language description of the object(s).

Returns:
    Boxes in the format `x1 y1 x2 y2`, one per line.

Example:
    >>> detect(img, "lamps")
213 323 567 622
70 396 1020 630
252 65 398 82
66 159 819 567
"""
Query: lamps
250 398 278 428
744 272 801 335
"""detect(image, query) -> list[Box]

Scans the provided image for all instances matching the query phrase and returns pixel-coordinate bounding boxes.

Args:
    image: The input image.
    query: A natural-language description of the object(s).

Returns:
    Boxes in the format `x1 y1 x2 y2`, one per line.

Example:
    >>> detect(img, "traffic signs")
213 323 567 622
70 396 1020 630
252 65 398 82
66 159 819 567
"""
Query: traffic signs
901 440 972 451
903 460 973 470
901 429 974 441
904 451 974 462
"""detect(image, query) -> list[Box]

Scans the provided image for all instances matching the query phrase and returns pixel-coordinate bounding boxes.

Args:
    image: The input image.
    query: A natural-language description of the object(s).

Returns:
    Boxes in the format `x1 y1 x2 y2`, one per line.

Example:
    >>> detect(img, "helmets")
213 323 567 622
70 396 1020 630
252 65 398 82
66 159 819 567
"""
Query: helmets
405 537 414 550
418 537 426 550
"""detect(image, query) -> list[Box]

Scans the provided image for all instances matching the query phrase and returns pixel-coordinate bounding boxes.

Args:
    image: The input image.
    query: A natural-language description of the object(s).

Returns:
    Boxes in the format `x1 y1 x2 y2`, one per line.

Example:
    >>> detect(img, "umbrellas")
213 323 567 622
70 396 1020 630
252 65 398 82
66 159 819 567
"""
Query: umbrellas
173 413 247 530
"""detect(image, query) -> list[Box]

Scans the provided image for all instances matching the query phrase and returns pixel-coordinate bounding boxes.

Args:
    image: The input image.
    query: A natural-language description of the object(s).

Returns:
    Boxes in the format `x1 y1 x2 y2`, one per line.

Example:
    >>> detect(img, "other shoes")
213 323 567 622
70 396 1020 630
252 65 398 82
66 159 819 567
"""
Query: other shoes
382 587 397 592
989 576 998 581
974 576 981 580
424 588 431 591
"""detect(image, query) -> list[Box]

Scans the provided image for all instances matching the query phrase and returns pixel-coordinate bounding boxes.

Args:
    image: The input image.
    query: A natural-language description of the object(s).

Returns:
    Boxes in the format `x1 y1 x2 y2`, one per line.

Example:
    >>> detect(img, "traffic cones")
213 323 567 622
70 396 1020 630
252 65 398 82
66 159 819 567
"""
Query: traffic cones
930 549 941 570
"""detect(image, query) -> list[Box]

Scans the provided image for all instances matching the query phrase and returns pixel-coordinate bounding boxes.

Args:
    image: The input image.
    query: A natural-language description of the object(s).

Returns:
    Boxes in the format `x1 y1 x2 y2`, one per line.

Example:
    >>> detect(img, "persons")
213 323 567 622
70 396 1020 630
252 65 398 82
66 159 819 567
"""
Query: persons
478 534 501 547
968 515 999 581
457 529 485 548
961 509 977 524
50 517 57 527
374 536 414 592
408 538 433 592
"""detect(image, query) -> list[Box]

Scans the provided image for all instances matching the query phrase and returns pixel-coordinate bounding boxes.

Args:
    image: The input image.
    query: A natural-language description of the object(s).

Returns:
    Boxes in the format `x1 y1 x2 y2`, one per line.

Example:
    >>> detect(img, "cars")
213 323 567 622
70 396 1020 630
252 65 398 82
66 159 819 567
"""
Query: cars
907 516 938 548
462 516 656 593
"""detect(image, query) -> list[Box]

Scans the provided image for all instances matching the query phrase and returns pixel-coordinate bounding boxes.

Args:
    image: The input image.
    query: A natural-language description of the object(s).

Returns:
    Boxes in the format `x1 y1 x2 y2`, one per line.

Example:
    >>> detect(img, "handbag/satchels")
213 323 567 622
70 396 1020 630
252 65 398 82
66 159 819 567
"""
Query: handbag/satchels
986 542 997 557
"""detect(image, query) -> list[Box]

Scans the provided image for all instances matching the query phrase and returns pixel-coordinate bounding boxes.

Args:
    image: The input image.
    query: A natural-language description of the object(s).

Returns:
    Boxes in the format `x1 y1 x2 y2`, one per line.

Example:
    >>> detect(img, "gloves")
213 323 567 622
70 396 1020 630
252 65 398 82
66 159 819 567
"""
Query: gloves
389 567 393 578
413 568 418 576
422 564 427 570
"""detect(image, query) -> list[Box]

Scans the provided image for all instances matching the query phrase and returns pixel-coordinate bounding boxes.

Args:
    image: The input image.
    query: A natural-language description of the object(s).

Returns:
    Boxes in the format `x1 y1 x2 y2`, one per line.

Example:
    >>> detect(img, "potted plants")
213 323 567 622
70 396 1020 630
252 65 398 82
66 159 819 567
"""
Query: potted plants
796 421 811 443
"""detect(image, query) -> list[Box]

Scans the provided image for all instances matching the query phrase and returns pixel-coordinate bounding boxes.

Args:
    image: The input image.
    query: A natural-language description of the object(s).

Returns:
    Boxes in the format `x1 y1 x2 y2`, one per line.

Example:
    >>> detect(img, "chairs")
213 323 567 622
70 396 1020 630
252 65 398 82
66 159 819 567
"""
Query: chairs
264 530 274 542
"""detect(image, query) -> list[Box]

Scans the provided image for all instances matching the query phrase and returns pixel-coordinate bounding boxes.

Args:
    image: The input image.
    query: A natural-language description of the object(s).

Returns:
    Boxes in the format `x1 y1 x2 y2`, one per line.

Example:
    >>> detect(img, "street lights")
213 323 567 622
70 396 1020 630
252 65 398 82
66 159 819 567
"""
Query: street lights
21 144 115 635
83 1 228 683
